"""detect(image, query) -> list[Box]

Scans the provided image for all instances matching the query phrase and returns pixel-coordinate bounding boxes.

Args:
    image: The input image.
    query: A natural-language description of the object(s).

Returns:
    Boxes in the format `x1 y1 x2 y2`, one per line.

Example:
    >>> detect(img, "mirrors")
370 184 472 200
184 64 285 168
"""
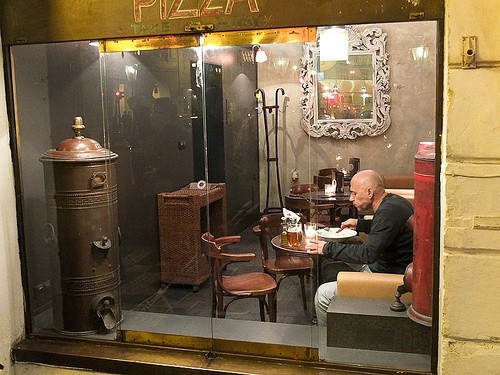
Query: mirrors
299 24 391 140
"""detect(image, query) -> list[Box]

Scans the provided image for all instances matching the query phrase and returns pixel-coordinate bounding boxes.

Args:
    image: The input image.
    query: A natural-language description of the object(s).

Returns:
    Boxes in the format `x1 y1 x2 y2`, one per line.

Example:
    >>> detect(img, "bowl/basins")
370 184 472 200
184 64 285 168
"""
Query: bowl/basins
316 228 357 243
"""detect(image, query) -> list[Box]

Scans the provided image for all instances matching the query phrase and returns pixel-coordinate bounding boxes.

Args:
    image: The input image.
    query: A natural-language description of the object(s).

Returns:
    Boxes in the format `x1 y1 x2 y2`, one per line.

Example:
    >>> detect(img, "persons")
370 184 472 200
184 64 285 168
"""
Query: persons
305 170 414 328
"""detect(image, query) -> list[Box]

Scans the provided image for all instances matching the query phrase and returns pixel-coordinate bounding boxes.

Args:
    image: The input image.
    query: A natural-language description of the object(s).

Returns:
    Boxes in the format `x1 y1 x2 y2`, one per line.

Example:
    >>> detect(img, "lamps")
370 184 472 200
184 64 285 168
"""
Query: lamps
252 44 267 63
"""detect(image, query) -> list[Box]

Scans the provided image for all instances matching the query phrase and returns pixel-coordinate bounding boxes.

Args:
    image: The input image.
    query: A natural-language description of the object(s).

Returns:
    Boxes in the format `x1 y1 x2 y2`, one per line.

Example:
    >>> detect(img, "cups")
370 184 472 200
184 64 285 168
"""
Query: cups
286 221 302 247
304 223 317 242
335 172 345 194
324 184 335 195
281 222 287 247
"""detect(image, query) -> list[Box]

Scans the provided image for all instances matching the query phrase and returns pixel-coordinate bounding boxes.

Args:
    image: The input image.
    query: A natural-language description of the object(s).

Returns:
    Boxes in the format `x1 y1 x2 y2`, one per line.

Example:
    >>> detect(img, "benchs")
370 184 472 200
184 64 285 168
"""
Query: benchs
328 173 415 298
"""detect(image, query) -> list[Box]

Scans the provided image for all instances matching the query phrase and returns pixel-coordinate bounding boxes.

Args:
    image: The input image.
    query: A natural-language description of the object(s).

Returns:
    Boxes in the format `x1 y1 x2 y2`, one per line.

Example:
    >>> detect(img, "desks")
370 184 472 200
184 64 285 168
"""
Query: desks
300 190 358 230
314 172 350 189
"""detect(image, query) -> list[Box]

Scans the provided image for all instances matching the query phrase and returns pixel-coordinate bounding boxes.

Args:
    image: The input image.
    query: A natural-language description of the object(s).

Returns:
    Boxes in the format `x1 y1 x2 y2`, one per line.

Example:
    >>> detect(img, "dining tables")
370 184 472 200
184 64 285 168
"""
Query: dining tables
271 230 365 322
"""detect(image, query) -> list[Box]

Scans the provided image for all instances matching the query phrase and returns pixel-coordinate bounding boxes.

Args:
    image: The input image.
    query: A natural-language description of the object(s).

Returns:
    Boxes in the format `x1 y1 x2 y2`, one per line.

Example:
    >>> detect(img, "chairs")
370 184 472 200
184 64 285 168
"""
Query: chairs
201 158 340 323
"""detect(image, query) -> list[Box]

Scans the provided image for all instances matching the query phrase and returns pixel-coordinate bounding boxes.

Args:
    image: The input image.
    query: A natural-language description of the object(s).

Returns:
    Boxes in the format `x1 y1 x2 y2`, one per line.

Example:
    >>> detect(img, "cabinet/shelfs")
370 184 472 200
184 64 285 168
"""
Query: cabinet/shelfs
46 45 260 289
158 183 227 285
